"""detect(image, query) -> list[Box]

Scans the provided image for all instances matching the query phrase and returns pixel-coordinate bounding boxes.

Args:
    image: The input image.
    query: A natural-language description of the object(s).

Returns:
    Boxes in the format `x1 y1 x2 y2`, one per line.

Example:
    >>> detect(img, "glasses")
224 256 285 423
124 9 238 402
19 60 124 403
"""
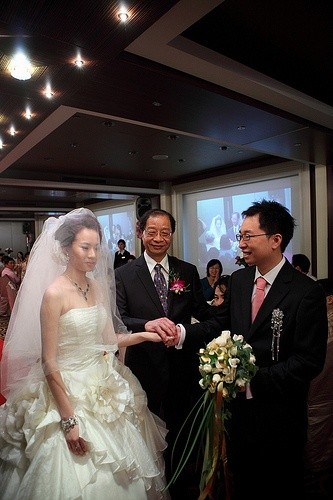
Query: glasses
144 228 172 238
235 233 268 241
231 218 237 220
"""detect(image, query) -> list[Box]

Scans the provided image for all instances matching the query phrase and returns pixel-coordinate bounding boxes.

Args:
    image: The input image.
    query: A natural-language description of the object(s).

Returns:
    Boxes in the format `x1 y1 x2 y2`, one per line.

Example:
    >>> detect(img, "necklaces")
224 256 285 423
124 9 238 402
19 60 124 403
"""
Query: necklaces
67 276 90 300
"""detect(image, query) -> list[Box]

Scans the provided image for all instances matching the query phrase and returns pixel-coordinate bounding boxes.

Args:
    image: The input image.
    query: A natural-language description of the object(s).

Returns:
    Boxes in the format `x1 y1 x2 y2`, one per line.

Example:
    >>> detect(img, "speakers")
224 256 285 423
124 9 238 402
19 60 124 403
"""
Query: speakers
136 196 153 220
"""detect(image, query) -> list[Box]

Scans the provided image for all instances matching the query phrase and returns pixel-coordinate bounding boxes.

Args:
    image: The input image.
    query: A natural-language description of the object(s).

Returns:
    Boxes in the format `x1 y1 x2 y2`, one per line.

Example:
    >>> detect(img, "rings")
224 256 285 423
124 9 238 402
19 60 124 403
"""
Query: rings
75 446 79 450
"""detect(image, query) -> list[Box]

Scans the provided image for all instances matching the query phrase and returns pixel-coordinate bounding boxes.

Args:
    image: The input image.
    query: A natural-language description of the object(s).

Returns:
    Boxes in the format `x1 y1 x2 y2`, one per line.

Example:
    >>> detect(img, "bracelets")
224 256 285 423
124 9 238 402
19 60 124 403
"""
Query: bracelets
61 418 77 431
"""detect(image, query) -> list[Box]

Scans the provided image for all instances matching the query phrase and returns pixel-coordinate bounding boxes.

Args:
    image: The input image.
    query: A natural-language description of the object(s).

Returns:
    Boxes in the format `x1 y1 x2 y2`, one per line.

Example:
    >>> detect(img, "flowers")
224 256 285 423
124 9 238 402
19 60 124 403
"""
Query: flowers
164 330 256 495
169 270 192 295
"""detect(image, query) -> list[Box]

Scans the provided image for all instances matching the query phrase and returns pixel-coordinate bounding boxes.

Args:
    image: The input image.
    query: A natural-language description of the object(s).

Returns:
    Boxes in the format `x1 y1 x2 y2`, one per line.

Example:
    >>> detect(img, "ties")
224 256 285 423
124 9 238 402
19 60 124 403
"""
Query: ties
251 278 267 323
153 265 168 317
235 227 237 233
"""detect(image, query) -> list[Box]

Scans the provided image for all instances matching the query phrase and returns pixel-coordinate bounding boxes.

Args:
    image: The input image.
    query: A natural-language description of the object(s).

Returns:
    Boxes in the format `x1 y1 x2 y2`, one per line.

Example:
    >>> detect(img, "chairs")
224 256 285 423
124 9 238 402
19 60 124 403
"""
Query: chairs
0 274 18 318
308 338 333 468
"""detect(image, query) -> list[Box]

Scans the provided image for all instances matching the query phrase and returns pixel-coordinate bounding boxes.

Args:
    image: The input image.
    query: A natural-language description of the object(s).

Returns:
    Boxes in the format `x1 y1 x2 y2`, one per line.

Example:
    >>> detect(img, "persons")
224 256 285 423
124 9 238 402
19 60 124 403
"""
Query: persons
215 275 232 307
0 208 177 500
0 252 30 270
114 239 130 269
167 199 328 500
127 255 136 263
292 254 317 282
314 278 333 337
114 208 221 500
200 259 222 301
2 257 22 290
